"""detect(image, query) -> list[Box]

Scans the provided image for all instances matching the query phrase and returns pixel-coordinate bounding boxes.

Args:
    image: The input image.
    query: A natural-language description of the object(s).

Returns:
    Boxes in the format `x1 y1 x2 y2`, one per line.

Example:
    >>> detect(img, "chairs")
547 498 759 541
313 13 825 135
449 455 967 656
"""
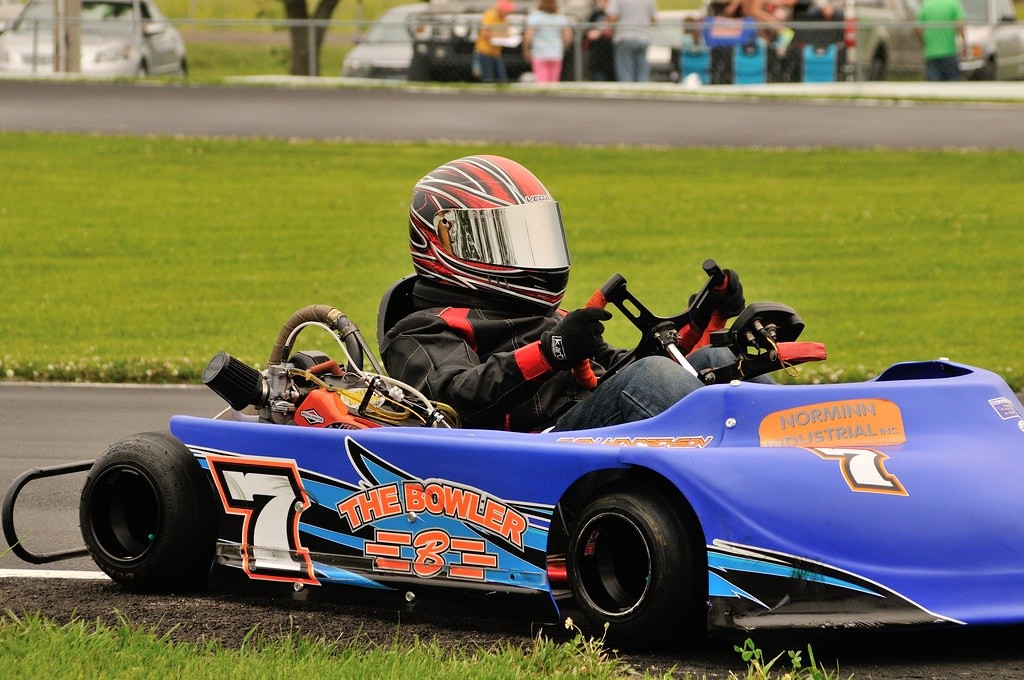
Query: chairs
669 34 846 82
374 273 418 346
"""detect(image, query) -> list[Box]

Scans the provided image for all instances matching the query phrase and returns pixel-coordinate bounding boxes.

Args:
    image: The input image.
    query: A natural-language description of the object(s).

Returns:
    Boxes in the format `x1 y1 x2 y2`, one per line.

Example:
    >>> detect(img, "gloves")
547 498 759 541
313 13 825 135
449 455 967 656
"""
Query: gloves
540 307 613 371
688 269 745 330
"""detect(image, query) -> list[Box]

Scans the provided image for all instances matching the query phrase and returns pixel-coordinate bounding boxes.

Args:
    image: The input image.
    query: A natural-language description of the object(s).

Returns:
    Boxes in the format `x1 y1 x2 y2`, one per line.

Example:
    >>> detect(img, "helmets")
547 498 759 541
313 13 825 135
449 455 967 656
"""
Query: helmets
408 154 572 315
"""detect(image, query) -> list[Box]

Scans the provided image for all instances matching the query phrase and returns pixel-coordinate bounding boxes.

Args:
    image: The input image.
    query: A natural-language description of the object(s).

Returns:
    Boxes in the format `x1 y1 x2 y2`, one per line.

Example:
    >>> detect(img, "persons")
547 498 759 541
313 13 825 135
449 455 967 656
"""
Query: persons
475 0 970 84
379 155 777 432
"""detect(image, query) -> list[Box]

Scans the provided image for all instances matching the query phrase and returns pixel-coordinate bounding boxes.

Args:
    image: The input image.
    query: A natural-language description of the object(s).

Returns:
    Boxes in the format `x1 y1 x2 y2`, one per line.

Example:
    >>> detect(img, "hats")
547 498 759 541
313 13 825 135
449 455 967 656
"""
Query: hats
497 0 515 14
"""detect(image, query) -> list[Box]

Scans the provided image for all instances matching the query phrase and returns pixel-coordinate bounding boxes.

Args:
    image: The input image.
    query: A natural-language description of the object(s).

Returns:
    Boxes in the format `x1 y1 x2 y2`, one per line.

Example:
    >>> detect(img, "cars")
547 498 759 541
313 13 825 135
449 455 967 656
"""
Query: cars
641 10 707 80
846 1 932 80
0 0 190 79
408 1 610 85
936 0 1024 78
344 0 432 81
